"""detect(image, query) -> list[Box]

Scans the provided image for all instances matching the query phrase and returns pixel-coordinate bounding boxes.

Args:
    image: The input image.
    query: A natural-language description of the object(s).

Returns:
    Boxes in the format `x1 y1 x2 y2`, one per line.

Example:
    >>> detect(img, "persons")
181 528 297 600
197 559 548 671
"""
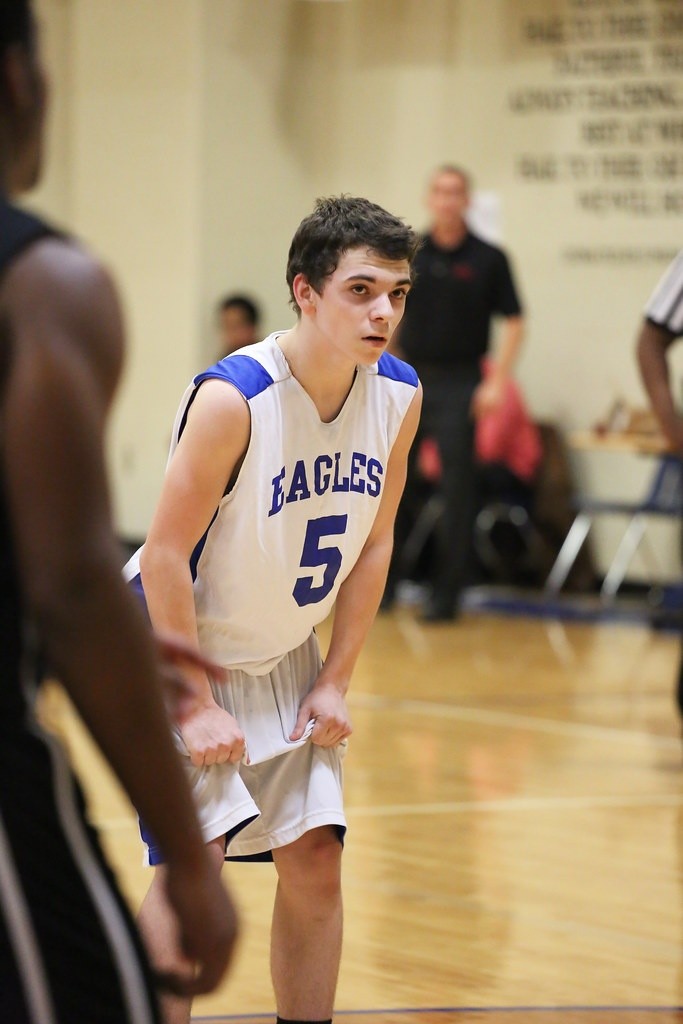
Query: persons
0 0 238 1024
415 322 542 590
121 197 422 1024
385 167 523 622
212 298 259 361
636 247 683 715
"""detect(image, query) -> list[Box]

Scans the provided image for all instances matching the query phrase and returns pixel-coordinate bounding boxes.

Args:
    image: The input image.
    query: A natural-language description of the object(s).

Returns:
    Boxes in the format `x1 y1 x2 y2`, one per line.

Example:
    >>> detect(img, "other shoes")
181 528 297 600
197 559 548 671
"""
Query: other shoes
417 604 458 621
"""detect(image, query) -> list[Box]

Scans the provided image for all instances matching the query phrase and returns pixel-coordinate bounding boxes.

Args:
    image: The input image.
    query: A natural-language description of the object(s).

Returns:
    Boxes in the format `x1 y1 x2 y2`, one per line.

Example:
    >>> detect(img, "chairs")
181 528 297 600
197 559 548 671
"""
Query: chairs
540 449 683 599
